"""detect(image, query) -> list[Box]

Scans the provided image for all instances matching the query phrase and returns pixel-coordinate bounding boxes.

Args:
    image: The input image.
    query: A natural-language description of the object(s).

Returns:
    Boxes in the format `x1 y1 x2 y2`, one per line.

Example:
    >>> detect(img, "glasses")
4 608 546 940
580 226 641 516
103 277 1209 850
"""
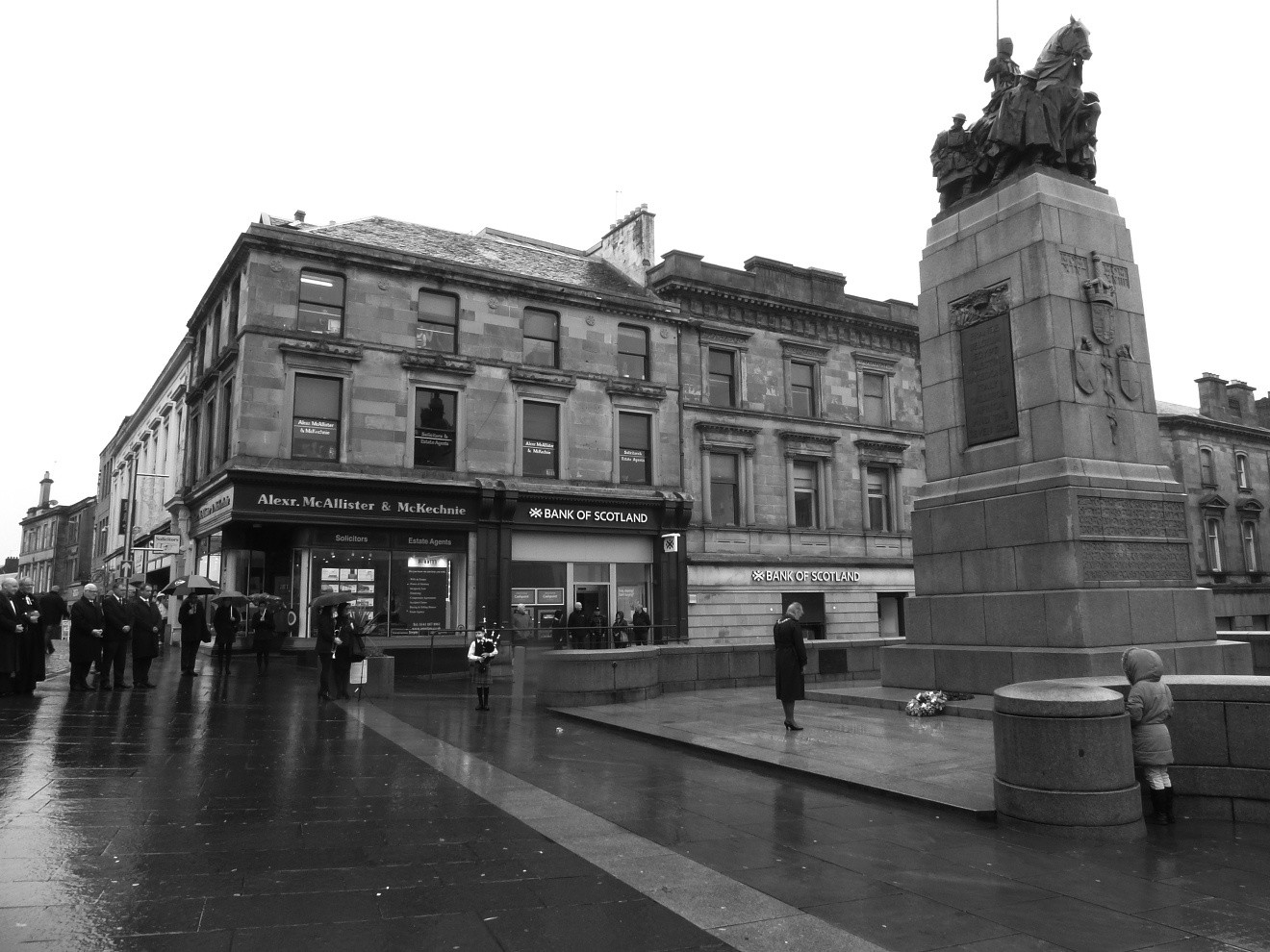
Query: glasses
86 589 99 593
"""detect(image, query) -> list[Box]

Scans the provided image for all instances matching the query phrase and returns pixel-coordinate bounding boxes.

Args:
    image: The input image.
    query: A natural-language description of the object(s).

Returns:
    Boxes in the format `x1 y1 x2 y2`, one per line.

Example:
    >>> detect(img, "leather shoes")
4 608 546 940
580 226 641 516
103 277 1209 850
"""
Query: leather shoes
114 679 131 689
71 682 96 690
101 680 111 691
190 669 199 677
181 669 190 676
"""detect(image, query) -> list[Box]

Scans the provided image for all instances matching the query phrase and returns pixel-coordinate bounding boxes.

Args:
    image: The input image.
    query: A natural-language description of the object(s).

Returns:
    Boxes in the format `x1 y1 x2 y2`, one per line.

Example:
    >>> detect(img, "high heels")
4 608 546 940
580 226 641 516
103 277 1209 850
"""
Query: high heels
789 721 803 730
784 721 791 730
318 691 332 700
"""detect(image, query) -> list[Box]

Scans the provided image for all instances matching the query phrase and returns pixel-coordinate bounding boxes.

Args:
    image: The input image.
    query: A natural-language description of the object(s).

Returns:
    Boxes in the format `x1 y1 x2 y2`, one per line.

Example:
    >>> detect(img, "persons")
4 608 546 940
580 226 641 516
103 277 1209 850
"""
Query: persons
1121 647 1177 826
773 601 808 731
467 625 499 712
382 588 402 622
509 601 651 657
0 571 358 701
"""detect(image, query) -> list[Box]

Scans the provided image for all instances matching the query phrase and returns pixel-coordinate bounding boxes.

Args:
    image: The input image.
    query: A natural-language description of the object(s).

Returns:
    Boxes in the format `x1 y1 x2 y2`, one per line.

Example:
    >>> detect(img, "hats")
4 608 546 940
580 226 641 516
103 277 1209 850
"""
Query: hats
593 606 601 611
474 625 485 632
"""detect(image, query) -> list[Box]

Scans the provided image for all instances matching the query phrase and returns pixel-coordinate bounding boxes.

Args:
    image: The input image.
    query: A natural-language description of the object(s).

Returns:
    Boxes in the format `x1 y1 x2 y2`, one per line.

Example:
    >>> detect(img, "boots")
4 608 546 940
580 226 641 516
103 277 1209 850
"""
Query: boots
1164 784 1174 823
263 655 269 678
225 655 232 676
476 687 484 710
484 687 490 710
1144 786 1169 824
217 654 224 675
256 656 262 678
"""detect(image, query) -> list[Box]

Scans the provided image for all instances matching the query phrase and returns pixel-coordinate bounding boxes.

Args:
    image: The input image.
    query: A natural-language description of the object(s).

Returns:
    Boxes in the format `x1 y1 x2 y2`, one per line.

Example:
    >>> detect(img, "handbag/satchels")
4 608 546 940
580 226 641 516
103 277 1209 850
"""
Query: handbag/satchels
620 631 629 642
